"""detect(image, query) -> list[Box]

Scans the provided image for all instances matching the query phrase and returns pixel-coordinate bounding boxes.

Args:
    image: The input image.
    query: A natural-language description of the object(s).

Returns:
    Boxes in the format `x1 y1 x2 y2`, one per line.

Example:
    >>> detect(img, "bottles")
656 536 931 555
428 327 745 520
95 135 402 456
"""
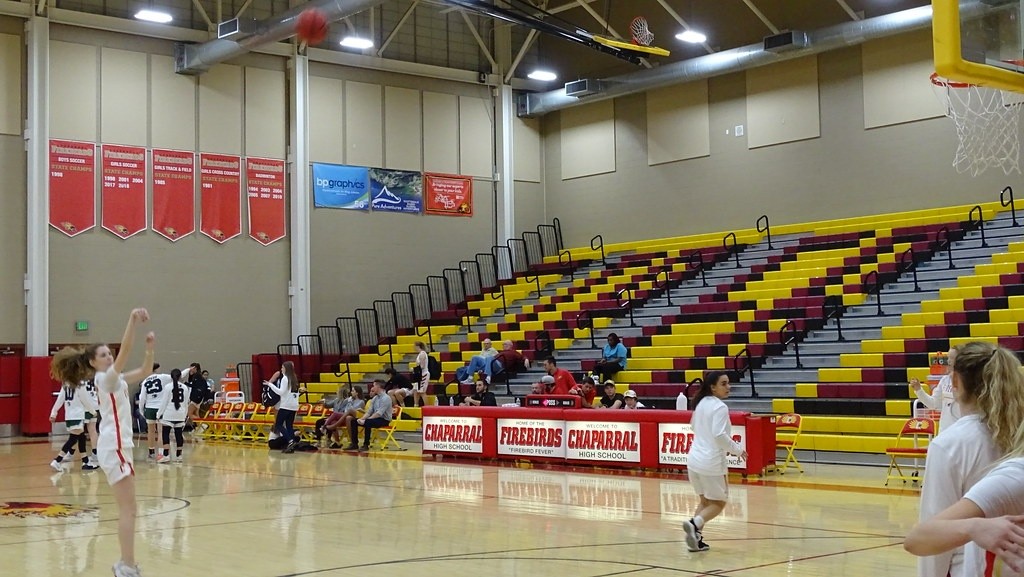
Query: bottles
675 392 687 410
434 397 438 406
516 398 520 407
450 397 454 406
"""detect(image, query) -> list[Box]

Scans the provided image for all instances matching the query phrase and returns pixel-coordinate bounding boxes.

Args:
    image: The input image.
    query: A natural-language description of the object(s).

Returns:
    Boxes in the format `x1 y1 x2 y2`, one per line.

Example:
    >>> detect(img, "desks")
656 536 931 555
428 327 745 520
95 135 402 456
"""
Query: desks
423 406 776 480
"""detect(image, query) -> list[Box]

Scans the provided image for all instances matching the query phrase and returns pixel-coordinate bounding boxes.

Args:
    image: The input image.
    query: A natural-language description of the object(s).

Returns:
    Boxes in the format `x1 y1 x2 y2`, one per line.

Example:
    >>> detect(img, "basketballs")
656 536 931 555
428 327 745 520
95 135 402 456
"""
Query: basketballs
294 8 330 46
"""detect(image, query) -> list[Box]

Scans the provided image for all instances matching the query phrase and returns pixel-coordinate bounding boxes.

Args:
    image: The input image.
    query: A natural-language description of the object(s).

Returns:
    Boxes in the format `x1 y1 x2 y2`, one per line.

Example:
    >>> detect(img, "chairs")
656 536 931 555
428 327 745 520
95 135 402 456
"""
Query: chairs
884 416 937 487
185 400 404 451
773 413 804 472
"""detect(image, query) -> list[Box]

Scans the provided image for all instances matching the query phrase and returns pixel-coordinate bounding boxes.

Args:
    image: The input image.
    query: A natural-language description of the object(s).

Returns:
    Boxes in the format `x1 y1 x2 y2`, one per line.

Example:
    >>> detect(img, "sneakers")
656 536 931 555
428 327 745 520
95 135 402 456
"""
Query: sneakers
50 460 64 471
683 518 701 552
157 455 171 462
113 562 141 577
82 460 99 469
687 542 709 551
155 454 163 461
172 454 182 462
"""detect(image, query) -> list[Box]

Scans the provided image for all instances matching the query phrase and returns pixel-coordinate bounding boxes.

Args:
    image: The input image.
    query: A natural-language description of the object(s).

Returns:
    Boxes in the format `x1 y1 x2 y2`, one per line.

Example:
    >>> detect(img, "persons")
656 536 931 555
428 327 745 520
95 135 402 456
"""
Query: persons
683 371 750 552
139 362 195 461
48 308 155 577
532 356 576 394
412 341 430 407
63 379 99 465
384 368 413 407
457 338 499 380
623 390 645 410
262 361 307 454
185 362 215 432
593 380 626 410
596 333 627 382
48 378 100 471
460 340 530 384
156 368 189 463
569 377 596 408
459 379 497 406
134 381 148 434
910 345 965 436
314 380 392 451
902 419 1024 577
917 341 1024 577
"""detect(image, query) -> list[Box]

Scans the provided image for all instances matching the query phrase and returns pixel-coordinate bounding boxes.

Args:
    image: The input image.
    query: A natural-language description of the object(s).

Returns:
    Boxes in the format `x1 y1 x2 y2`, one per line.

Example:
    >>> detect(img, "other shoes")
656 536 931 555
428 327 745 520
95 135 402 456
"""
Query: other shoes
147 454 155 461
330 442 342 449
357 446 369 451
63 451 73 461
197 424 209 434
91 452 97 461
343 442 358 450
282 441 296 453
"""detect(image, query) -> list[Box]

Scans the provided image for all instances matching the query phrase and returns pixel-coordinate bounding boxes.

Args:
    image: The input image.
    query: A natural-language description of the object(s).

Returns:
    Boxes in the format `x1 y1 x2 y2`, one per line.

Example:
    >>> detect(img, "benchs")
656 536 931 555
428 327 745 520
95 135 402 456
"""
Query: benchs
291 206 1024 452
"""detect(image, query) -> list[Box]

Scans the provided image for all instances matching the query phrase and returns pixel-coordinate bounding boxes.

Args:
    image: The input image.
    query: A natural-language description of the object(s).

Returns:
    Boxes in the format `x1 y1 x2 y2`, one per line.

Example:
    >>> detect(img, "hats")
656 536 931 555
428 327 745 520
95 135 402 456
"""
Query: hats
623 389 636 398
542 376 554 384
604 380 615 386
